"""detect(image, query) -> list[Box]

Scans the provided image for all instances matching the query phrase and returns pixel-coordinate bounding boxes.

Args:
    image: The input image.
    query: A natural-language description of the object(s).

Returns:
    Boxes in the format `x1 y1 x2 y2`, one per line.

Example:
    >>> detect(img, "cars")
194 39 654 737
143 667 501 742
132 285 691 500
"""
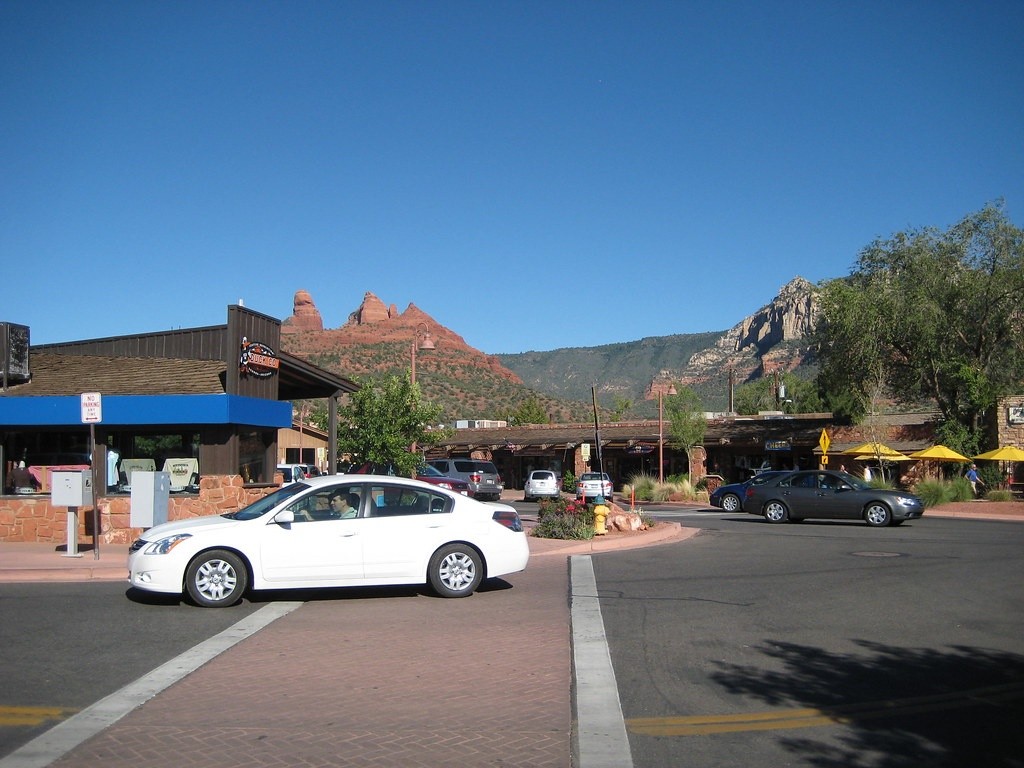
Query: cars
297 463 327 479
522 471 562 501
574 472 615 503
710 469 831 512
743 469 925 527
127 473 530 608
350 458 474 501
277 464 308 492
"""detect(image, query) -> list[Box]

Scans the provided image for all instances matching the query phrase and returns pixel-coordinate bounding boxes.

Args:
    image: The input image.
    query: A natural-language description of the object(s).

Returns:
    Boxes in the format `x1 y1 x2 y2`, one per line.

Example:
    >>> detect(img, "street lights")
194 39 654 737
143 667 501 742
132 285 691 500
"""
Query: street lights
658 378 677 486
410 322 436 480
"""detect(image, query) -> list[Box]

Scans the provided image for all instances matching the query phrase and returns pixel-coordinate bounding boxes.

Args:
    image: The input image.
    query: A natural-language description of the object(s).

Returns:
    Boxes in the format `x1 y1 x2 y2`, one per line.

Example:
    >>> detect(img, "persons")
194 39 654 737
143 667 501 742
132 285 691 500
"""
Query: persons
964 464 985 495
839 465 847 474
301 490 357 521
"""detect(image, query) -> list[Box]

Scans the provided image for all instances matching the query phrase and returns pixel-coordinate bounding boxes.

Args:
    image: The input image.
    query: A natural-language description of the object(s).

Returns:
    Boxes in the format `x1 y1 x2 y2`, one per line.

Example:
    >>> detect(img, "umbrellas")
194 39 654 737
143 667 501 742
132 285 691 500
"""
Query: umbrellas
908 444 973 483
844 442 909 481
969 445 1024 488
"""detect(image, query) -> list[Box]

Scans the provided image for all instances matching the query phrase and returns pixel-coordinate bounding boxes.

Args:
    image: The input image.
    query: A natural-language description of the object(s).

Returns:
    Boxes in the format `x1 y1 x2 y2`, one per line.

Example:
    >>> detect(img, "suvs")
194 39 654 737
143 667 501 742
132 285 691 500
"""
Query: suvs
424 458 503 501
